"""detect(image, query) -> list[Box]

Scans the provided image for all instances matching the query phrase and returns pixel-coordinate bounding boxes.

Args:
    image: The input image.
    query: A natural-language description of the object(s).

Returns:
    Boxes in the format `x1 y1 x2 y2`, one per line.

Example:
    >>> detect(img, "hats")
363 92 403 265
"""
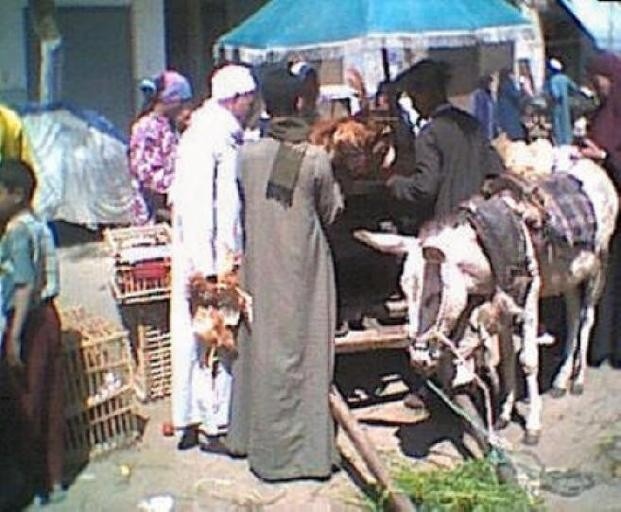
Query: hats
393 58 454 95
208 62 258 103
548 57 563 71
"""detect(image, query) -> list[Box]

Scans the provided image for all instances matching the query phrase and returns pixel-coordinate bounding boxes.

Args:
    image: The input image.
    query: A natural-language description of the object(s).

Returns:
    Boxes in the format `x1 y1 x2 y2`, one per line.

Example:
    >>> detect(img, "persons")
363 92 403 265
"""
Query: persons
0 159 69 505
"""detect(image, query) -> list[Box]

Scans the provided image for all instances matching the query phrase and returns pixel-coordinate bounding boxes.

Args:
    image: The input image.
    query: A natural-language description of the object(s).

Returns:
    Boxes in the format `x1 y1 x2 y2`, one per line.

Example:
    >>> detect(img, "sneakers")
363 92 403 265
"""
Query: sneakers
32 480 69 507
176 427 230 456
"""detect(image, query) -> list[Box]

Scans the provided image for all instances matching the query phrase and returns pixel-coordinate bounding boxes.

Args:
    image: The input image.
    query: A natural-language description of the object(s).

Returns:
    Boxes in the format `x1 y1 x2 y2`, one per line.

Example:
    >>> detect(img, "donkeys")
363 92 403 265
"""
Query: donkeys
351 154 618 444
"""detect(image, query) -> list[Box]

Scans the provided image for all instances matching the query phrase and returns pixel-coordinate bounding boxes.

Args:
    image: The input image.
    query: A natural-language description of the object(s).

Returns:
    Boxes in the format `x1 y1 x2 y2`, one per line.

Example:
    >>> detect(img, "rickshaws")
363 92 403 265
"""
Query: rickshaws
325 165 536 511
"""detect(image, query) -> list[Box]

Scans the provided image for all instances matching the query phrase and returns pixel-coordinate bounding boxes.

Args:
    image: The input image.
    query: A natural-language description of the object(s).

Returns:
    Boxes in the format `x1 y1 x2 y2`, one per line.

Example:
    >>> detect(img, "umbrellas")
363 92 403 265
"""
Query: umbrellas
213 1 544 120
